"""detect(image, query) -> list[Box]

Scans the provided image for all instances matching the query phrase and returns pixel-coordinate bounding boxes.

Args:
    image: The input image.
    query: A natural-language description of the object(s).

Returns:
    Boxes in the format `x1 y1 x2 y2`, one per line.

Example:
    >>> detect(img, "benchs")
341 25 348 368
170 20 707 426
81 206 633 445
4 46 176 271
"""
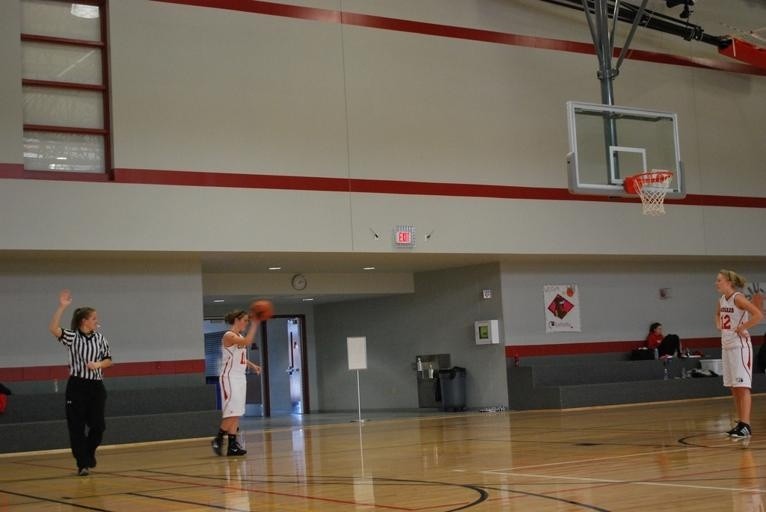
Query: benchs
509 345 765 412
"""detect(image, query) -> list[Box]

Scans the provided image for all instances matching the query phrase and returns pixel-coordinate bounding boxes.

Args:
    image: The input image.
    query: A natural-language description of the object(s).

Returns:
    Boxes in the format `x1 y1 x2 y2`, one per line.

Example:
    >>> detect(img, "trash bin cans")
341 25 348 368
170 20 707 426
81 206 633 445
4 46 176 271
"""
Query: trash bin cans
439 368 466 412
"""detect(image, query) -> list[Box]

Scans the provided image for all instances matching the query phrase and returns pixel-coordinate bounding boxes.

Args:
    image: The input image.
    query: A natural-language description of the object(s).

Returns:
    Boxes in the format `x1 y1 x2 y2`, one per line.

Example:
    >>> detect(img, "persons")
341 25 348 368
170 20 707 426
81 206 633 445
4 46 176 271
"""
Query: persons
715 269 763 438
50 288 112 475
646 323 688 360
211 309 262 457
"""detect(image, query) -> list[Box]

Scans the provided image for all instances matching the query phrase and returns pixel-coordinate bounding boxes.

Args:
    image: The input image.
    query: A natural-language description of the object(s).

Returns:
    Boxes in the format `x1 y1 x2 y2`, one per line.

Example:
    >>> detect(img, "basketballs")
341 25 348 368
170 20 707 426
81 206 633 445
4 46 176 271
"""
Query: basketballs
251 301 273 322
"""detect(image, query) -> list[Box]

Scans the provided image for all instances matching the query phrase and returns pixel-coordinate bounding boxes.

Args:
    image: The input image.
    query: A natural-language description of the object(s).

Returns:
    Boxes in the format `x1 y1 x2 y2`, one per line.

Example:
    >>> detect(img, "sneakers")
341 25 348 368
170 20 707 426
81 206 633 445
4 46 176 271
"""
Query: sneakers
78 457 96 475
725 421 752 438
211 439 247 456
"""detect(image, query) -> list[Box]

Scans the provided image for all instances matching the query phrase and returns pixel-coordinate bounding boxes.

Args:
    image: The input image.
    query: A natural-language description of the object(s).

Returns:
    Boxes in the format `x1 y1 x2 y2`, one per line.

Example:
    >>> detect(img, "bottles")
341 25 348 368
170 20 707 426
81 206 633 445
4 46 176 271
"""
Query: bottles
655 348 659 361
664 368 668 381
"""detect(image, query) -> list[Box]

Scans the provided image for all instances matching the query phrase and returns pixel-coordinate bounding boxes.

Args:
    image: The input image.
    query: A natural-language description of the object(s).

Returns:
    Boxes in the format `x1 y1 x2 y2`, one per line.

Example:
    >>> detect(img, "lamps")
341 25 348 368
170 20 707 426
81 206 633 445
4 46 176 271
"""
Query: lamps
345 335 369 423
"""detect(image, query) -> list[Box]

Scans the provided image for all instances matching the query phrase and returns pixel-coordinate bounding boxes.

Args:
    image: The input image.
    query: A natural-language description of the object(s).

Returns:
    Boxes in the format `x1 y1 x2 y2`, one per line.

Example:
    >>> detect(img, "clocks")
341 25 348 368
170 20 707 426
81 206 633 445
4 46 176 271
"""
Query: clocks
291 274 307 290
